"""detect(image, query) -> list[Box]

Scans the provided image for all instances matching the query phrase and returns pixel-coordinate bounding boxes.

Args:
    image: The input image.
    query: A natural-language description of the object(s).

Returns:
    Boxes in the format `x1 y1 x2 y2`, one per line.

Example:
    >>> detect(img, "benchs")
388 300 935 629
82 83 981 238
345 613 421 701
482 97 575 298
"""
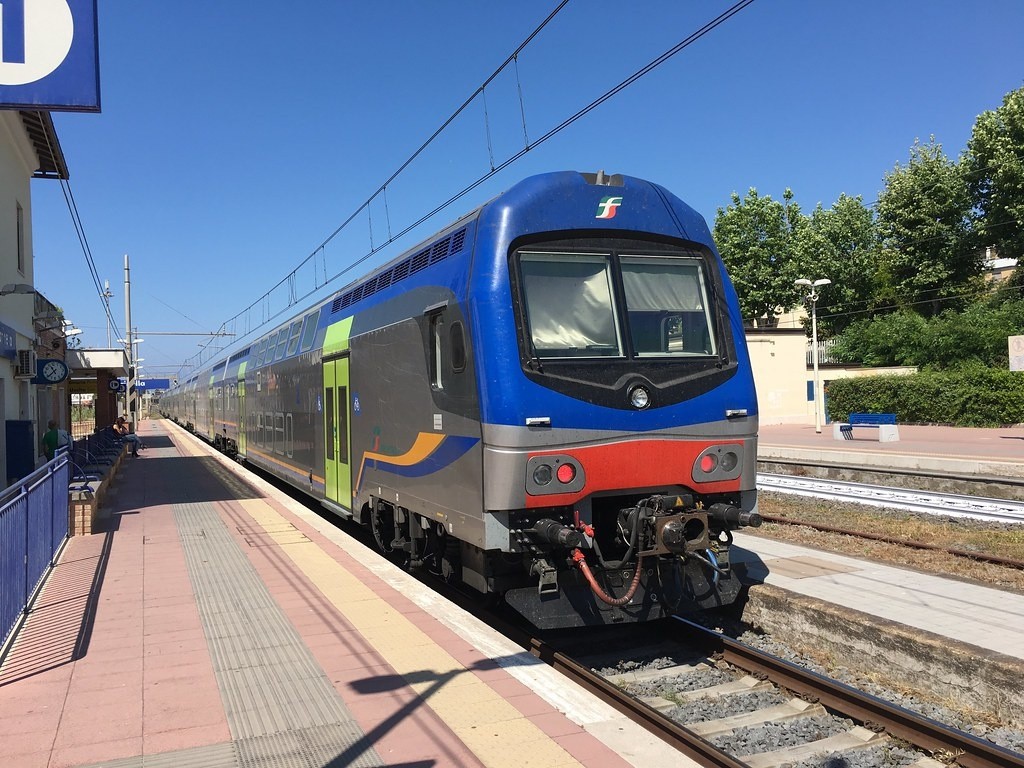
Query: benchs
55 426 128 536
833 413 900 442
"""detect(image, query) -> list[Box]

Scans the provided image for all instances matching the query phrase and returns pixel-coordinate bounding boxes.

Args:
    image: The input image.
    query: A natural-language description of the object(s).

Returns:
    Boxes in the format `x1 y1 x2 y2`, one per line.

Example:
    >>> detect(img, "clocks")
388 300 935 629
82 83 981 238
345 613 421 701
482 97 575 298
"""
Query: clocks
38 359 69 384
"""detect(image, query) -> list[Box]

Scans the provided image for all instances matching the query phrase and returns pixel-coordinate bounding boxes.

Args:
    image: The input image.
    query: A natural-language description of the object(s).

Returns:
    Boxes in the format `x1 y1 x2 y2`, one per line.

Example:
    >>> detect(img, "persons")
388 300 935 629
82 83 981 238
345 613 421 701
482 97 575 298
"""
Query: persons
43 420 72 469
113 417 148 457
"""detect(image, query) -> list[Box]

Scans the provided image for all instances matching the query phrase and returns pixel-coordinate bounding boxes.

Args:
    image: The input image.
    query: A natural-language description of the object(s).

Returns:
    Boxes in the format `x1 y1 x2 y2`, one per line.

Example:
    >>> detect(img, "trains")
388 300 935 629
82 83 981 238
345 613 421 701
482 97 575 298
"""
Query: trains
151 171 770 631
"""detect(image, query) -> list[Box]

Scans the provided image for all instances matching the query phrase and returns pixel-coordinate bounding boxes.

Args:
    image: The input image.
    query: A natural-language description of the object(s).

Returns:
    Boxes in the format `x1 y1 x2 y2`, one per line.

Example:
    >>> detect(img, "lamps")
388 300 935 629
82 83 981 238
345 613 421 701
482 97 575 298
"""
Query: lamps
33 310 83 343
0 284 37 295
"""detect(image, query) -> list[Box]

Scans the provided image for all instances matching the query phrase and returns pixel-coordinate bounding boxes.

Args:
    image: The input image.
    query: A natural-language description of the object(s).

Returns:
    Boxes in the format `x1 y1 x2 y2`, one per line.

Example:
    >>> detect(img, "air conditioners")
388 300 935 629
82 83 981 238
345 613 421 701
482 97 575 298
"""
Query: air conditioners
14 349 38 376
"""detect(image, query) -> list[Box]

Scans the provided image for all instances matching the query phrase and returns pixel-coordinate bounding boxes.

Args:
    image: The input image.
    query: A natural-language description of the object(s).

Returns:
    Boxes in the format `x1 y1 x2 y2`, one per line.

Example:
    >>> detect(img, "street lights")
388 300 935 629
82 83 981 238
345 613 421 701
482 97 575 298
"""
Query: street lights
794 279 832 434
132 357 144 431
116 339 145 344
